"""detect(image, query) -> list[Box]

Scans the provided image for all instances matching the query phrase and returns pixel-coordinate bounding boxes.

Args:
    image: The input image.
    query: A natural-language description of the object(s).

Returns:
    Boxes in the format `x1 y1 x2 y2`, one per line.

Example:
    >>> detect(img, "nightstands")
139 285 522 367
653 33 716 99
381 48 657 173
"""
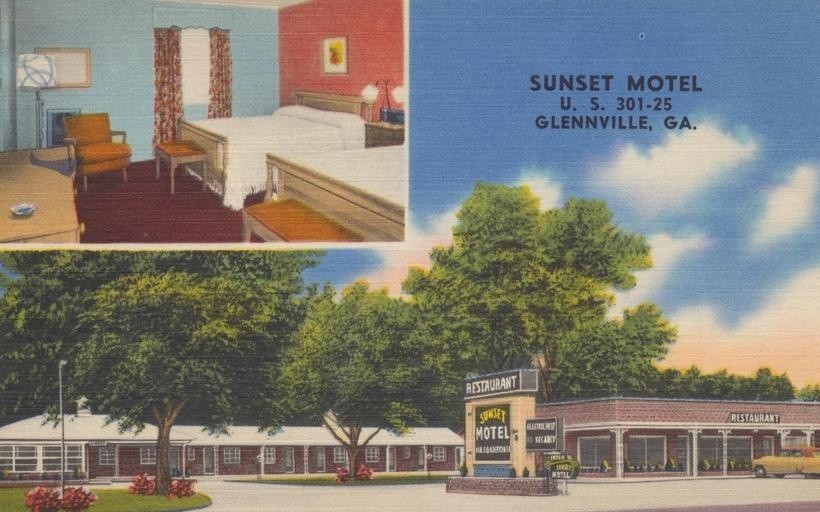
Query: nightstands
364 114 405 149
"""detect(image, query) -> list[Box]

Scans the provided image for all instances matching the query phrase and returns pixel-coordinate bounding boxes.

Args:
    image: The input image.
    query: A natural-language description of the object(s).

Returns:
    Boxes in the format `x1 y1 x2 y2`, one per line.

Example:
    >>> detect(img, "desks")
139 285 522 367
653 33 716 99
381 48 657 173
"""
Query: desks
0 142 82 244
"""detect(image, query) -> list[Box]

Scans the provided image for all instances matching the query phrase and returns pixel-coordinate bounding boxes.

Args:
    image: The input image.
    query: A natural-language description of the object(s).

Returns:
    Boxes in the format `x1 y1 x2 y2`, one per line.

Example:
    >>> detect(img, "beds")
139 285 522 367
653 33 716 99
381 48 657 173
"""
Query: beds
264 139 407 242
177 92 372 211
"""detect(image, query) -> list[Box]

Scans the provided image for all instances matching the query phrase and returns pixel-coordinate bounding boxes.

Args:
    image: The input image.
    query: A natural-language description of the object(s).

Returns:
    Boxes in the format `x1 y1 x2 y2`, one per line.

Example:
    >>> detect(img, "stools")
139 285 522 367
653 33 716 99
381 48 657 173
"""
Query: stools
239 197 364 241
154 139 209 196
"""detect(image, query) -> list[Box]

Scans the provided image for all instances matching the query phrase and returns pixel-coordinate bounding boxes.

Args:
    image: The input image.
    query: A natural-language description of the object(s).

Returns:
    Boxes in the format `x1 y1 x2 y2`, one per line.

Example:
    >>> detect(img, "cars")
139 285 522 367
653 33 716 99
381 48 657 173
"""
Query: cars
752 446 820 478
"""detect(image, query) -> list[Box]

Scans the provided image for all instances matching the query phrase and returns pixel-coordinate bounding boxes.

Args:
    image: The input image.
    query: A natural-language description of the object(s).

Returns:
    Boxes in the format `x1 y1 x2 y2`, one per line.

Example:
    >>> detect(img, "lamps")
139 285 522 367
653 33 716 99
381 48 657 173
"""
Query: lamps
15 53 58 149
358 77 405 122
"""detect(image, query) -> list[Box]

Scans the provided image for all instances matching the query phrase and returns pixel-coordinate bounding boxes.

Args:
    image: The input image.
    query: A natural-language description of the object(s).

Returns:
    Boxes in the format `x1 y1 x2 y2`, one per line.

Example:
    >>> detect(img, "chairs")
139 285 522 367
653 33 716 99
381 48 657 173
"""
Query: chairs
61 112 132 194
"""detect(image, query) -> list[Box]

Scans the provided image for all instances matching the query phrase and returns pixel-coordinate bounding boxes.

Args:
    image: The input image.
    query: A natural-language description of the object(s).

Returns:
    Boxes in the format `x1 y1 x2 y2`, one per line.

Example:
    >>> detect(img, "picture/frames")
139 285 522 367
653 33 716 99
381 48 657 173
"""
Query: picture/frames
34 44 90 90
45 108 81 148
321 36 349 76
